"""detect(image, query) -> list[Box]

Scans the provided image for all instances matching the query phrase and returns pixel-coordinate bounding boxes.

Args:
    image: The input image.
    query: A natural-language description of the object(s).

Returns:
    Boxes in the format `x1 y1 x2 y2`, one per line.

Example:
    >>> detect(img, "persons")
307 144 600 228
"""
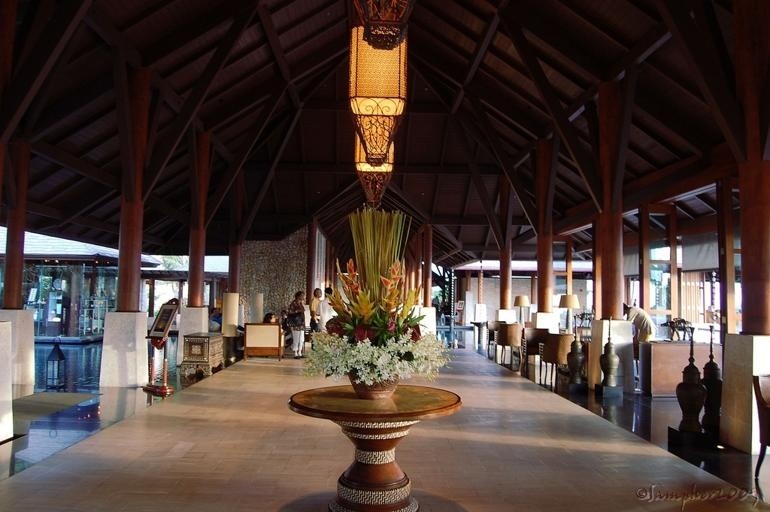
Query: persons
623 302 656 360
210 307 222 331
432 296 439 313
263 287 338 358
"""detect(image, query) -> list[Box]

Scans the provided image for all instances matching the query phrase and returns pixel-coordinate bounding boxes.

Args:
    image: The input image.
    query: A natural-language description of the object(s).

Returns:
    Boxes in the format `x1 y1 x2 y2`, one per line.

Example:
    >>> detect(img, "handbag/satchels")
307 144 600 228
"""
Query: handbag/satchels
286 312 304 327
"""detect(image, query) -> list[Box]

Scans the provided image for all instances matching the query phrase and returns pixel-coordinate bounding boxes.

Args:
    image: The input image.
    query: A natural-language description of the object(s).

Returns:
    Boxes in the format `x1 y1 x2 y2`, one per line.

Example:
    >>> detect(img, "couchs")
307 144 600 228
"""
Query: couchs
244 322 285 361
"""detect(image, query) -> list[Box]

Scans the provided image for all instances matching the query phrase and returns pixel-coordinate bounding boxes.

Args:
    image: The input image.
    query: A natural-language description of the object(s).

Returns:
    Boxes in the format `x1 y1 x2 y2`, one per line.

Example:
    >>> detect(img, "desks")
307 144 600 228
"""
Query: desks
289 385 459 511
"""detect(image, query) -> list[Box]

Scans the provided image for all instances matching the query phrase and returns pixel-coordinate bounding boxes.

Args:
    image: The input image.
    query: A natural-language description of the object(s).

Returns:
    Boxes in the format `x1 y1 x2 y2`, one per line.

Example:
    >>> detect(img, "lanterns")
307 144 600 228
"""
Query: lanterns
349 28 405 201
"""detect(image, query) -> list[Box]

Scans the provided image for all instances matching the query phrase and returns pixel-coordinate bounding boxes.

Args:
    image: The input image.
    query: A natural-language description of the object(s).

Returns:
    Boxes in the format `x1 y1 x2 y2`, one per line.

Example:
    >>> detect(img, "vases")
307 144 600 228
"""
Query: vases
347 362 400 398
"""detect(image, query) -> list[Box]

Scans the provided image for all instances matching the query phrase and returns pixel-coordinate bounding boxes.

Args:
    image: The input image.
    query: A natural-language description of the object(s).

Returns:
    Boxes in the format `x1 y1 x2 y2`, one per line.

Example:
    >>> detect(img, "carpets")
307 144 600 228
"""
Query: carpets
13 392 100 426
281 490 466 511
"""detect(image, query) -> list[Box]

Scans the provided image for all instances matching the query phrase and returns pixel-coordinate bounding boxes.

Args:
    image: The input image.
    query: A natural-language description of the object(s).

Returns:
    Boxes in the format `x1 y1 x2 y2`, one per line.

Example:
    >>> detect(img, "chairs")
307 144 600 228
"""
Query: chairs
487 320 573 391
751 371 768 483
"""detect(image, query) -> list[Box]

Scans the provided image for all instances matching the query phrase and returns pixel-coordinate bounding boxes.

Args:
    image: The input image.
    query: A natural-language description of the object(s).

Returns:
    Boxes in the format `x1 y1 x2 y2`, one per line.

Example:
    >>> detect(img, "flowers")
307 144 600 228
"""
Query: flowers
301 209 450 383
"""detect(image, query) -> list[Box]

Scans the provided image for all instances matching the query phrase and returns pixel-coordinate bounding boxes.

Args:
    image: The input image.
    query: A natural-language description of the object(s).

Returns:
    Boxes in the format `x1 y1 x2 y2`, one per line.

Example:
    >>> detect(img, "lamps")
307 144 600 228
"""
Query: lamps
251 293 264 322
222 293 239 362
515 296 531 326
559 295 579 335
343 1 414 209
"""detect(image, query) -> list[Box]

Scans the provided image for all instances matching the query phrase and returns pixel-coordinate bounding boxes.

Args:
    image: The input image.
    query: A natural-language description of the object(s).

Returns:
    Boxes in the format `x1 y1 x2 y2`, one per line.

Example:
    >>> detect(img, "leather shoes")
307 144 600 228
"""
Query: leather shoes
294 354 306 359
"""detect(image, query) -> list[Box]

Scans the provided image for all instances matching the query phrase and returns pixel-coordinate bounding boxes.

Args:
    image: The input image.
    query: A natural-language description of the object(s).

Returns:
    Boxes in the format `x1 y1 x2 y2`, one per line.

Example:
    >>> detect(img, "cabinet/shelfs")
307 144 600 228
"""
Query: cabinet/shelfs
183 333 223 378
